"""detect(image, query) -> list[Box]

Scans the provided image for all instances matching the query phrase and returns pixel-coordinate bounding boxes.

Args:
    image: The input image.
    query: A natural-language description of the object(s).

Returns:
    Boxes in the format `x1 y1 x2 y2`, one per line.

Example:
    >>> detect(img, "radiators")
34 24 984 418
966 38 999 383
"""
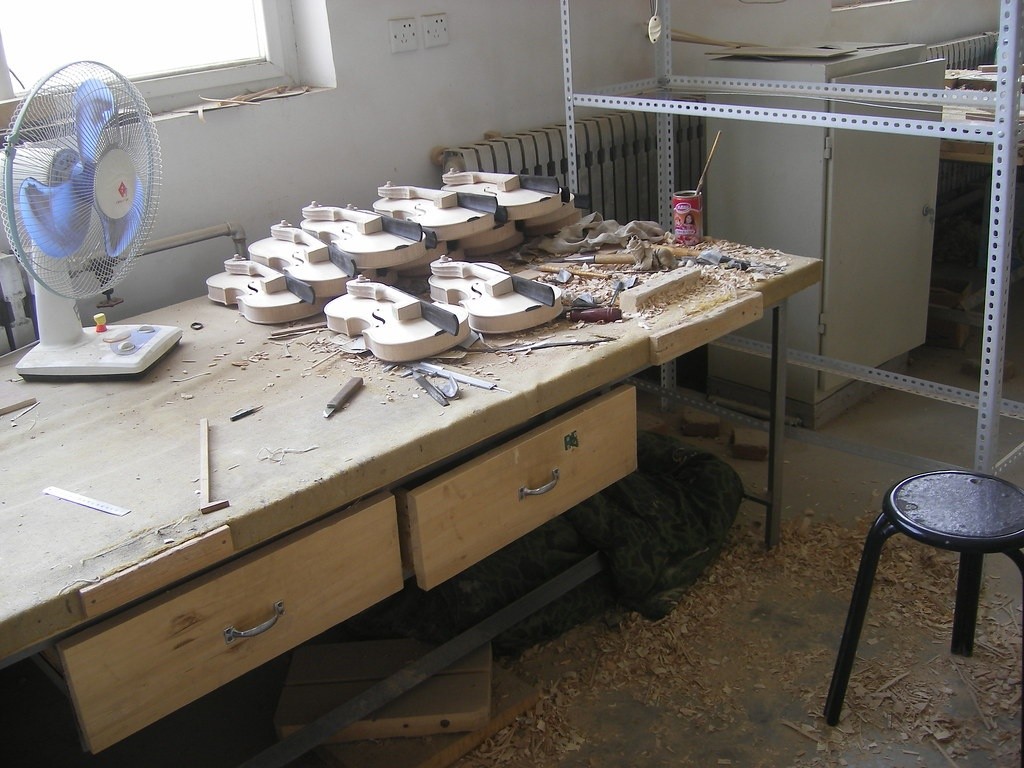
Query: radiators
442 31 1004 245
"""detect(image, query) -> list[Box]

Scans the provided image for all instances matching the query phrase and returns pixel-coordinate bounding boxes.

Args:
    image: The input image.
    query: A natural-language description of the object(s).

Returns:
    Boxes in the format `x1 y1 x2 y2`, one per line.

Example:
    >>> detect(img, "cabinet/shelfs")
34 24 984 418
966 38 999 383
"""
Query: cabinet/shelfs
699 42 945 427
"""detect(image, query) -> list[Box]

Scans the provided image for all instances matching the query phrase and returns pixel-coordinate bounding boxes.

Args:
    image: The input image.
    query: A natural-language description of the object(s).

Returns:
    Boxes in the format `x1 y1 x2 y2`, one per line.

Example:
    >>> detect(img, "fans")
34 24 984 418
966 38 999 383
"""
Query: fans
0 57 184 382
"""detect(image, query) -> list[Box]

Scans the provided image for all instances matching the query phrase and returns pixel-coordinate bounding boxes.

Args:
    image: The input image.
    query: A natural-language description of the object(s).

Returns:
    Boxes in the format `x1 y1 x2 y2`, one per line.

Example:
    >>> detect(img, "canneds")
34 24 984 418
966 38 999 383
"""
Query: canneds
672 189 703 246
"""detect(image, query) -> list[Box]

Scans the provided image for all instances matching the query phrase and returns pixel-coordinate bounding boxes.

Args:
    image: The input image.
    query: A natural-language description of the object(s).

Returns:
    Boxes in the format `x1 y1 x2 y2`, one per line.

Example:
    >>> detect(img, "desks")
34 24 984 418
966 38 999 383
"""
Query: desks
940 88 1024 166
0 235 827 767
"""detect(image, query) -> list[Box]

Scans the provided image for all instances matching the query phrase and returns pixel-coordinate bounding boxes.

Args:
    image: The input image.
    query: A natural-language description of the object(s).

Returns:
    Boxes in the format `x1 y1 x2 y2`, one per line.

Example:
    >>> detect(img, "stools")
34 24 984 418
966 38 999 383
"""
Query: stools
824 468 1024 767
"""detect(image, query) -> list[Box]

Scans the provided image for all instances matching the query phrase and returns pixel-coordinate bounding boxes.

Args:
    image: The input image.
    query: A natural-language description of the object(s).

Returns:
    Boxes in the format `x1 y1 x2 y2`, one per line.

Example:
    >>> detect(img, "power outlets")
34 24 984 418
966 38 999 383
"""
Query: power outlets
389 17 418 54
421 12 449 48
